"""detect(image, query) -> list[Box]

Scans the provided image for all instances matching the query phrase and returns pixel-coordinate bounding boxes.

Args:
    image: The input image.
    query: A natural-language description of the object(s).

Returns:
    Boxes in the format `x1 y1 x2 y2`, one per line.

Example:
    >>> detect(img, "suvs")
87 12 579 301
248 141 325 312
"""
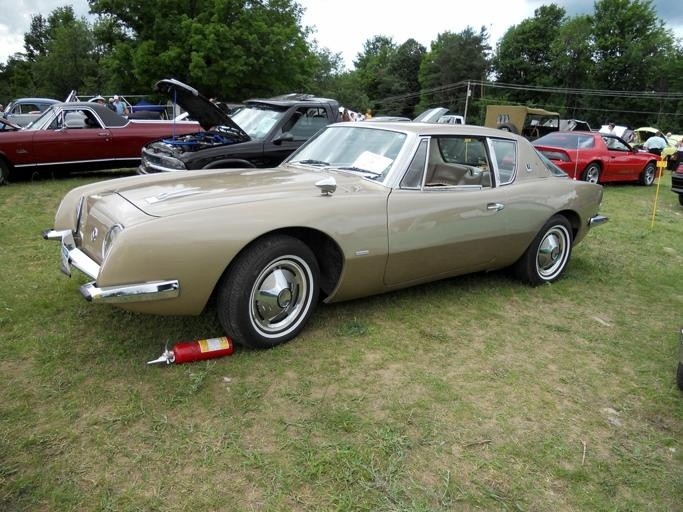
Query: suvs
136 78 340 173
484 104 559 143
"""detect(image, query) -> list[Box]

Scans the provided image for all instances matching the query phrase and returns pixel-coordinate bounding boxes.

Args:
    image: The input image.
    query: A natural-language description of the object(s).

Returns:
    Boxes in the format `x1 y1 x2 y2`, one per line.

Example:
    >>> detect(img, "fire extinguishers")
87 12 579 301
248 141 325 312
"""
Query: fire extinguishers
145 335 234 366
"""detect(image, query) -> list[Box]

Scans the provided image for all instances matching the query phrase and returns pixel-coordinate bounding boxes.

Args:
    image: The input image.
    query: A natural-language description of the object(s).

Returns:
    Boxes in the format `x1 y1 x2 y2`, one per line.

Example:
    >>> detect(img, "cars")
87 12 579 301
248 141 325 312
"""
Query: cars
1 98 63 126
636 127 683 168
531 131 663 187
0 102 199 186
43 122 602 346
670 158 683 207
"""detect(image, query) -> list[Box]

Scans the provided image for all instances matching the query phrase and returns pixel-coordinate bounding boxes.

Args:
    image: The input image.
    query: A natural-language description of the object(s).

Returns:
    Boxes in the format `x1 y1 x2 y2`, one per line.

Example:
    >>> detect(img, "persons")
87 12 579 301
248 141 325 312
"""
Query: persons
644 131 666 156
97 93 129 116
340 106 372 122
606 123 617 149
